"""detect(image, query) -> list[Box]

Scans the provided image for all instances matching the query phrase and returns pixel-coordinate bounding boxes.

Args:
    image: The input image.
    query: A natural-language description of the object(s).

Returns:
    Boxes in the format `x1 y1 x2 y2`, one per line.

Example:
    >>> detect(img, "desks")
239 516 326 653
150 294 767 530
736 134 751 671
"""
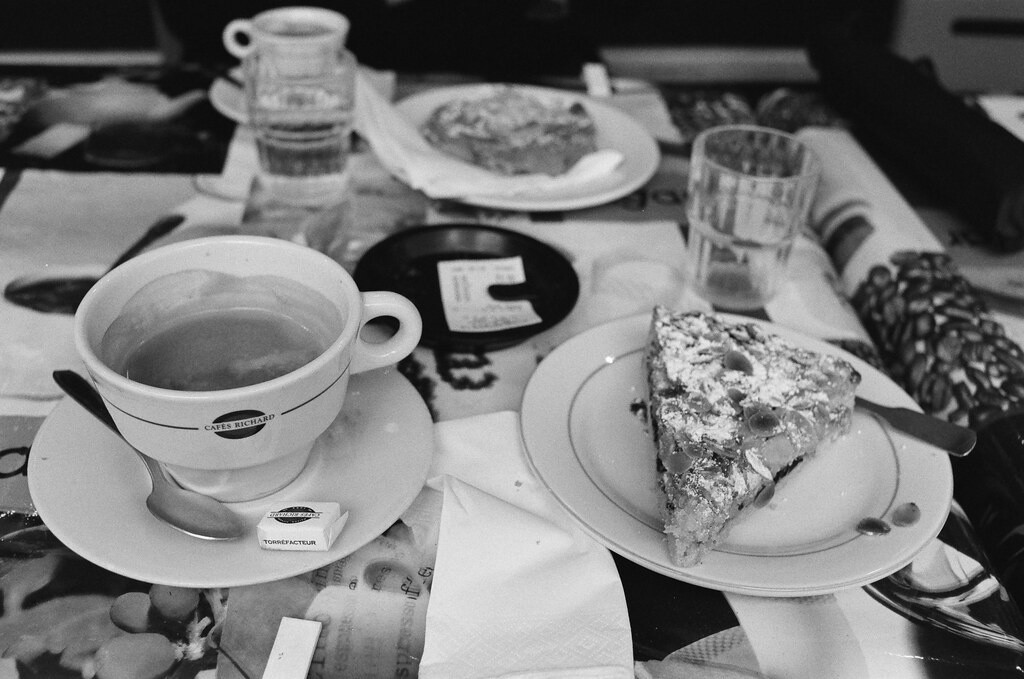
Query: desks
0 50 1024 679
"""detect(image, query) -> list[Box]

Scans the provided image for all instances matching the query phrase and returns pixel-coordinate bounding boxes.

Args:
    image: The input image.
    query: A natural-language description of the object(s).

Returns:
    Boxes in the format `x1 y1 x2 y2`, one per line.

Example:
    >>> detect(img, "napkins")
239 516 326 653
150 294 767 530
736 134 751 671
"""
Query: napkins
355 65 626 200
416 411 635 679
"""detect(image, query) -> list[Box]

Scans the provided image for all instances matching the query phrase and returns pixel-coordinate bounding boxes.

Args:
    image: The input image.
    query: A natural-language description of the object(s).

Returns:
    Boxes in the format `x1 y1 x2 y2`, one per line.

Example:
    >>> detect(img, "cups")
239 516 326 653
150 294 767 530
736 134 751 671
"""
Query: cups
688 126 821 307
223 8 351 61
242 46 355 206
71 235 422 501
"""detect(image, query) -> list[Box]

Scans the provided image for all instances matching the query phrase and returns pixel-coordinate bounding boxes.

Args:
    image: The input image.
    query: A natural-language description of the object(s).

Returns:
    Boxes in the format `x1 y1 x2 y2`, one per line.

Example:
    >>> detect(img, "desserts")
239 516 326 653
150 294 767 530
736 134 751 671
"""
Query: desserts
427 92 597 177
642 302 863 568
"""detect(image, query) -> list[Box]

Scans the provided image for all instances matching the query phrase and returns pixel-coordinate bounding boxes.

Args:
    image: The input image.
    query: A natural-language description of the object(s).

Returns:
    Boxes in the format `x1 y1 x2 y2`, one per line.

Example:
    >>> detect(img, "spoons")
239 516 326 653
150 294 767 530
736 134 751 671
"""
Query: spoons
52 368 243 538
5 214 185 349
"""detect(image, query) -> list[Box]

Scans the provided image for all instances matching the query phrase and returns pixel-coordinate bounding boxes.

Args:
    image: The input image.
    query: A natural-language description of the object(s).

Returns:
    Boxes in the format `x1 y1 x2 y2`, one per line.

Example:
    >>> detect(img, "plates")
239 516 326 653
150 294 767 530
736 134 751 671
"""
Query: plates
352 222 580 355
26 367 434 587
207 62 379 125
394 83 660 211
520 310 953 597
